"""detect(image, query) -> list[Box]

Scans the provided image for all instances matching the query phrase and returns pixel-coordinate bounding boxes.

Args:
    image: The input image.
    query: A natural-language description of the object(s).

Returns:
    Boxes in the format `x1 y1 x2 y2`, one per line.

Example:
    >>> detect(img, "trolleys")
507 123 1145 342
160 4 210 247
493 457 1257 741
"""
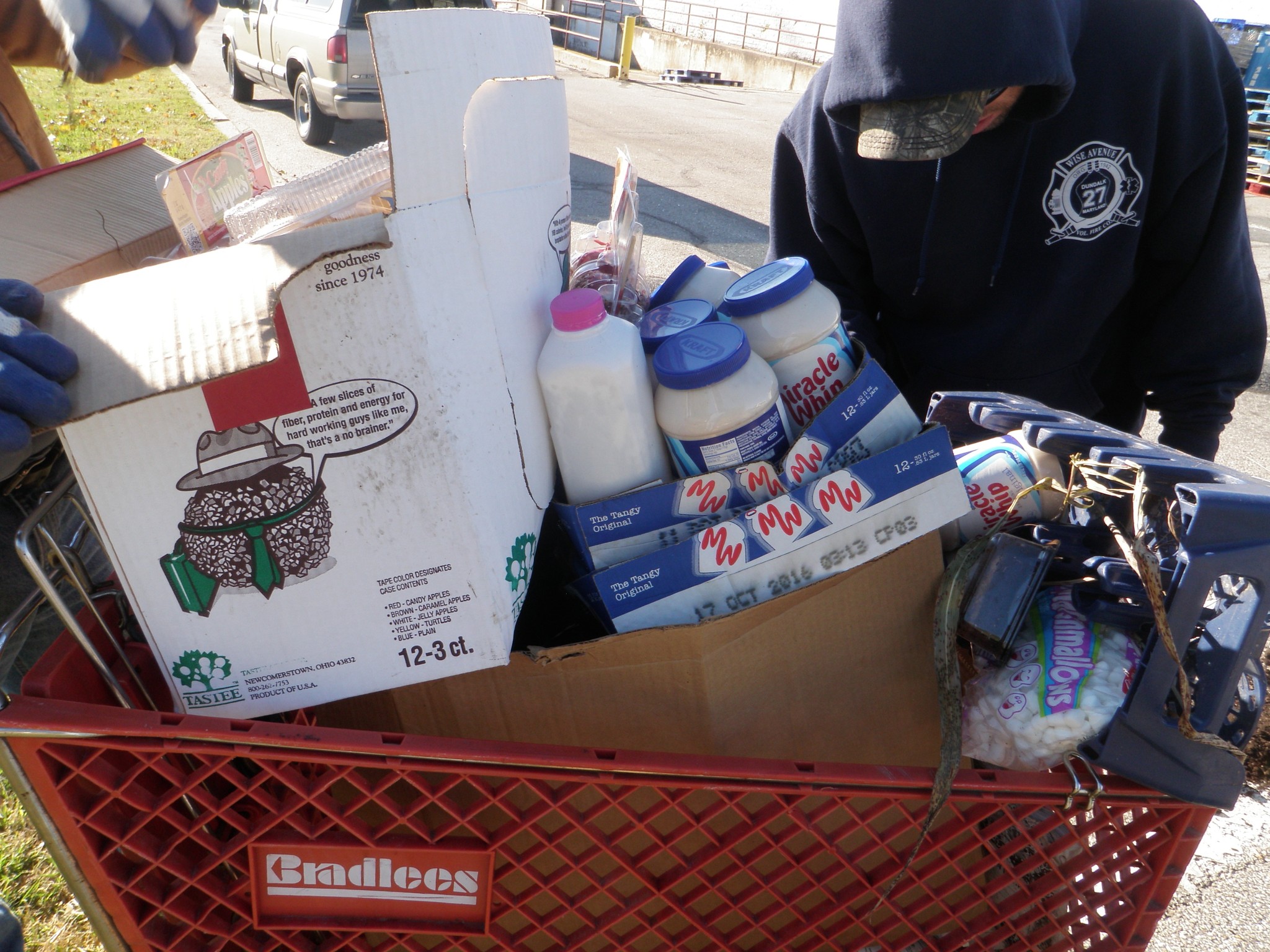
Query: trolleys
7 14 1270 952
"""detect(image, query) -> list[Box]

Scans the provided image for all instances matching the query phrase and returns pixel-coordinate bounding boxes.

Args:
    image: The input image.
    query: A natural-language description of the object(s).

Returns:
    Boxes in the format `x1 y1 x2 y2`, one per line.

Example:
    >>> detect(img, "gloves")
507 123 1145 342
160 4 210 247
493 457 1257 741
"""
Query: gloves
0 278 79 452
40 1 218 71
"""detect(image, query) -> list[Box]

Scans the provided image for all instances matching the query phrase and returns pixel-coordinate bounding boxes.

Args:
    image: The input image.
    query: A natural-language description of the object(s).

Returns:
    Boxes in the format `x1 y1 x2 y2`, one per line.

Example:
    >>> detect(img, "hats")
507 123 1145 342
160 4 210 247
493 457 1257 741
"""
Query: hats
855 89 1001 159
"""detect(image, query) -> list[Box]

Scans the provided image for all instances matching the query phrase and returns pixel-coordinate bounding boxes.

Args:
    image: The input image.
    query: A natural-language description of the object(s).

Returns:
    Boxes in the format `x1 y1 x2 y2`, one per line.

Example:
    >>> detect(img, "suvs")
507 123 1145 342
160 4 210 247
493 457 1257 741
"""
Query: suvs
215 1 494 143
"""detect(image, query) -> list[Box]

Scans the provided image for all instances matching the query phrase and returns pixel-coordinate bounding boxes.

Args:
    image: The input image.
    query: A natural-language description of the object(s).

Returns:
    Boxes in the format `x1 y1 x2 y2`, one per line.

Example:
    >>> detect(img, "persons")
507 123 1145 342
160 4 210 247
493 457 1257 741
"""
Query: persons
764 1 1267 463
0 1 221 451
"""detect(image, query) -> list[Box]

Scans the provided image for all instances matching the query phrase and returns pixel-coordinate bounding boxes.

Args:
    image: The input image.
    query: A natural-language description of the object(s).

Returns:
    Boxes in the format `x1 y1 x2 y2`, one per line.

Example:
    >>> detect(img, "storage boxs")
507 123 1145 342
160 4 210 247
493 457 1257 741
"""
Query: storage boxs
293 525 996 949
35 9 578 725
551 334 975 640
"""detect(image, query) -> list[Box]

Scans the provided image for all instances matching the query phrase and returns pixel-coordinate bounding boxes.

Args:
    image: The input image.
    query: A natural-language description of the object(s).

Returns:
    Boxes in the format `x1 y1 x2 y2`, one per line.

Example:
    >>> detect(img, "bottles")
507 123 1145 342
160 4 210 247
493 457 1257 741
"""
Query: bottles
573 220 647 325
636 296 718 391
536 291 671 504
725 255 856 439
932 430 1068 550
708 260 730 273
652 321 795 479
649 254 742 319
